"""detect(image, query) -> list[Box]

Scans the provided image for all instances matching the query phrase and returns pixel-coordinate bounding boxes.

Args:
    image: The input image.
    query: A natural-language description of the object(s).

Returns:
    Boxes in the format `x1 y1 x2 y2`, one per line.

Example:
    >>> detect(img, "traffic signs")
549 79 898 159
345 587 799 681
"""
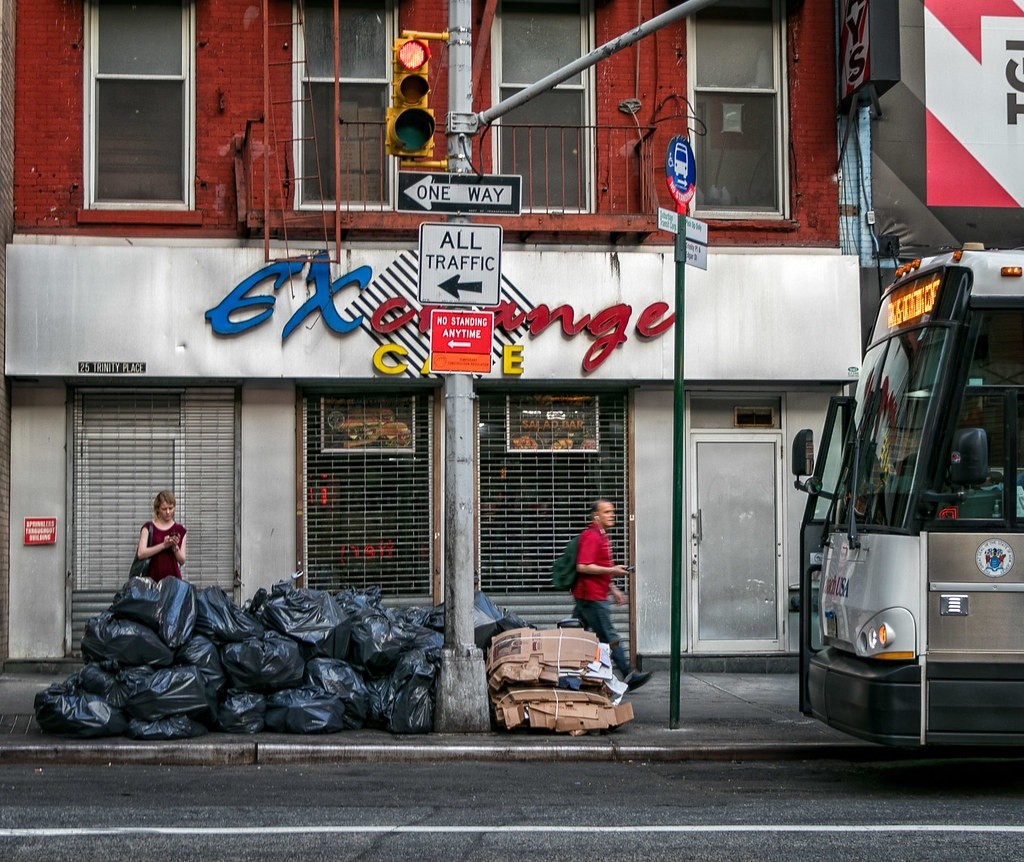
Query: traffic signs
416 221 503 309
394 170 521 217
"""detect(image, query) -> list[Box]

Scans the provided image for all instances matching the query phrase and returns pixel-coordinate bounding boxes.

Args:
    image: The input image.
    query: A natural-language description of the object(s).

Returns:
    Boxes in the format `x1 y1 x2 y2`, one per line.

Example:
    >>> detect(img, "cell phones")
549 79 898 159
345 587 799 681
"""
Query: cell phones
626 565 635 571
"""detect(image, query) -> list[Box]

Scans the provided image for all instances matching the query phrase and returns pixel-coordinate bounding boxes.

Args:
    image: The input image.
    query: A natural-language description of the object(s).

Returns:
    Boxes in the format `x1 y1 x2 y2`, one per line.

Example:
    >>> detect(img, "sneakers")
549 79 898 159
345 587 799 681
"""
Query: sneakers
624 669 653 691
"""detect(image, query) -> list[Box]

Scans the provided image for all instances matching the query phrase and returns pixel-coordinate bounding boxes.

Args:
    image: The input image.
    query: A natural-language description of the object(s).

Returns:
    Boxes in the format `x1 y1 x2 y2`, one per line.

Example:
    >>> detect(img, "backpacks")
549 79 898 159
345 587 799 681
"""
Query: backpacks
552 536 579 591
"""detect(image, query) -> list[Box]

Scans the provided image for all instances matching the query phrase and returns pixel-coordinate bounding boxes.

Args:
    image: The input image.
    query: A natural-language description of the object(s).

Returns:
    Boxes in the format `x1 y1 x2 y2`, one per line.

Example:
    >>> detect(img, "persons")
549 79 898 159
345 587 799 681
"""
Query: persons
138 493 189 587
554 500 654 694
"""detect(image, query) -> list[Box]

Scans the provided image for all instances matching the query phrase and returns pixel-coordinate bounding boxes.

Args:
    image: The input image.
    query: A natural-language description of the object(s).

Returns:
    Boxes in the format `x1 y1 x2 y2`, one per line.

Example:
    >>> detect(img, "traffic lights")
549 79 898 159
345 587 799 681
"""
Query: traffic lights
384 40 435 157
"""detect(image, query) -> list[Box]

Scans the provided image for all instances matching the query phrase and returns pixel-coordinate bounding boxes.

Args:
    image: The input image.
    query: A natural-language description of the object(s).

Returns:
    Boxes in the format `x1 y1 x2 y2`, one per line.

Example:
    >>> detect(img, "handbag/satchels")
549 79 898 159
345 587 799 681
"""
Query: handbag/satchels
129 521 153 579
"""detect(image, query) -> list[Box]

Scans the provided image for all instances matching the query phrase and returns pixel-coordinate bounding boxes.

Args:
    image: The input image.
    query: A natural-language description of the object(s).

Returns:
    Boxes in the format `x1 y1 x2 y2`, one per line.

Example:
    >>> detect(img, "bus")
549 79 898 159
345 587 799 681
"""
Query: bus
785 242 1024 772
